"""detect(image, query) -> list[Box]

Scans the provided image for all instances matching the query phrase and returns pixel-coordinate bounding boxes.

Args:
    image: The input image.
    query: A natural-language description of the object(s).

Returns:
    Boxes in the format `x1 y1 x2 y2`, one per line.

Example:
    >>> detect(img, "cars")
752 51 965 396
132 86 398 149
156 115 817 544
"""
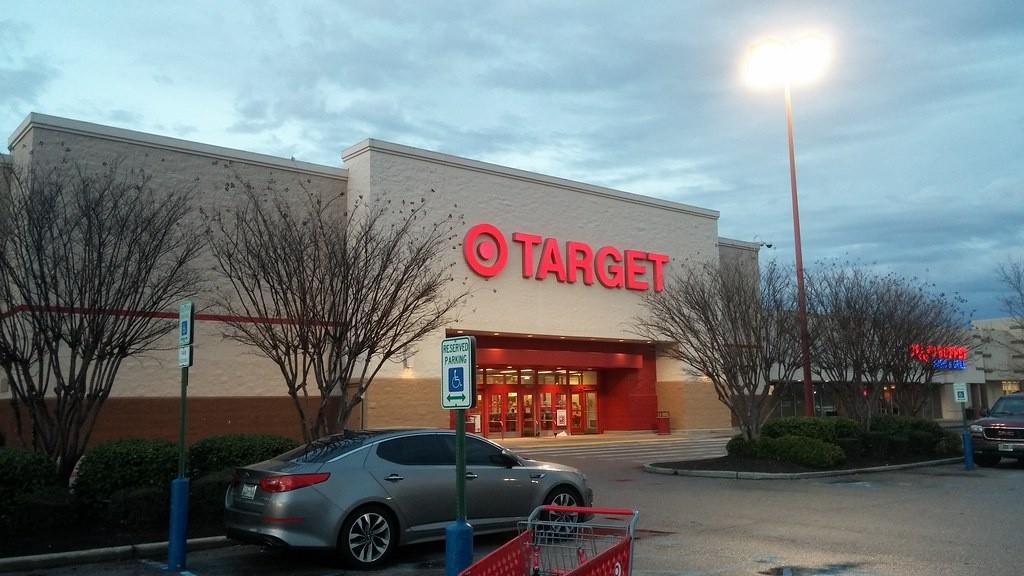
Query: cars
223 426 596 573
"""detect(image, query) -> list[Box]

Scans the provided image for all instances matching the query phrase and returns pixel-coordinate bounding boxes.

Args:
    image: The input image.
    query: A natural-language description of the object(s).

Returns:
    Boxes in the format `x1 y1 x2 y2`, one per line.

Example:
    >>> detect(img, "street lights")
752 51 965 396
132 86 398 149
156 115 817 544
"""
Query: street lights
746 26 837 416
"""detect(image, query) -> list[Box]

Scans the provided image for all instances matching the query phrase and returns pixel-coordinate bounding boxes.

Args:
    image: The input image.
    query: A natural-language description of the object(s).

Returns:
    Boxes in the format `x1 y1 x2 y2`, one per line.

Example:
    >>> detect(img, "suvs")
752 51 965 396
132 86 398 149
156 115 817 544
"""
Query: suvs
967 390 1024 469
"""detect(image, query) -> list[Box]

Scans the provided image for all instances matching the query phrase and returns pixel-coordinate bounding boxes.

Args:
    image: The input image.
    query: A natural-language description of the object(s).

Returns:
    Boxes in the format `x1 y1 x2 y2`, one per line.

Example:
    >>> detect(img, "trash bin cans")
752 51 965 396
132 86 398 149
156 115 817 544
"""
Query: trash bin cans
465 415 476 434
656 411 670 435
965 407 974 420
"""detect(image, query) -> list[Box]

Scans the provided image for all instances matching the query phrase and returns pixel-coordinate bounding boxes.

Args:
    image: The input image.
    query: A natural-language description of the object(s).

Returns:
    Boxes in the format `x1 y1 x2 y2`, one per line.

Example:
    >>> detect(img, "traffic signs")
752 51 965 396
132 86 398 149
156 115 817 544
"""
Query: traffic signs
441 336 476 409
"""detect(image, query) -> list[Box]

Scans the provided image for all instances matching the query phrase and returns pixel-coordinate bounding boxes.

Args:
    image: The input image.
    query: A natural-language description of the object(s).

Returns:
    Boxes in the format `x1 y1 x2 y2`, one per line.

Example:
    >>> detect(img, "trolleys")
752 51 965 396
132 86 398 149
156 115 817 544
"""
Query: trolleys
458 505 639 576
